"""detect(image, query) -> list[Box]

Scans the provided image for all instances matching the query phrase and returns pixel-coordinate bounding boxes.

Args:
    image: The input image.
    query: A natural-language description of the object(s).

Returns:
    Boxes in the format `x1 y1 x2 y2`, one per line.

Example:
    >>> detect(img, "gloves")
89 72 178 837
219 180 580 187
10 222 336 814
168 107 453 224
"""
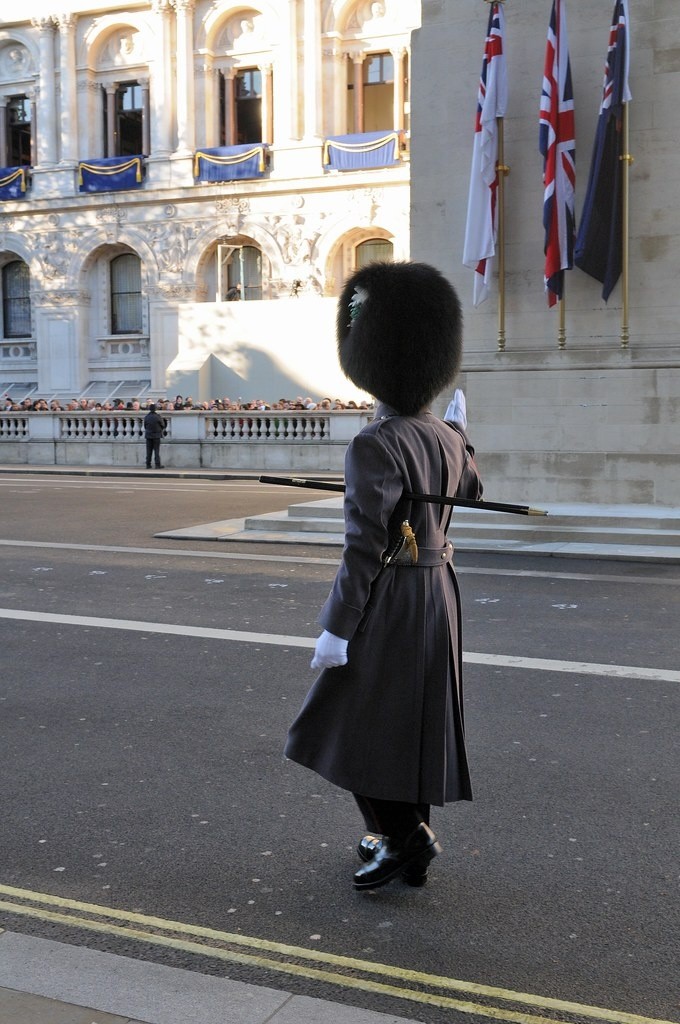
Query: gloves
310 629 349 669
443 388 468 431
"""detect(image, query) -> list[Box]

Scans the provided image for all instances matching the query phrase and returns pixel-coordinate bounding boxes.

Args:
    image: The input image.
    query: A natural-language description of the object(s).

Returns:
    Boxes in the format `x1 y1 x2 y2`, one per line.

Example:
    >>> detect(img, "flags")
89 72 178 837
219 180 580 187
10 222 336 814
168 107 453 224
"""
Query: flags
573 0 631 306
463 0 507 309
538 0 576 308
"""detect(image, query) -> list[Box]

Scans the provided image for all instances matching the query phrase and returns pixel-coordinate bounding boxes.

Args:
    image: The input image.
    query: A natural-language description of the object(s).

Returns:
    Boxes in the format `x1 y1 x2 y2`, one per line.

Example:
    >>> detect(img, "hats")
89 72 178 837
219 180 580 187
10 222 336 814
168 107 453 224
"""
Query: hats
336 261 463 418
306 403 316 410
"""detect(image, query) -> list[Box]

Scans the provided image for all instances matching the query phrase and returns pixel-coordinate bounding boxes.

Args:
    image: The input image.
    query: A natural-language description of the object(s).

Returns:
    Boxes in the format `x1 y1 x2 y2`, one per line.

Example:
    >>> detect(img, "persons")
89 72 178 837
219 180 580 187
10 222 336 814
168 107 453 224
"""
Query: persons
283 261 483 890
113 32 138 62
144 404 165 468
233 19 262 46
363 2 395 28
6 49 24 72
0 395 375 437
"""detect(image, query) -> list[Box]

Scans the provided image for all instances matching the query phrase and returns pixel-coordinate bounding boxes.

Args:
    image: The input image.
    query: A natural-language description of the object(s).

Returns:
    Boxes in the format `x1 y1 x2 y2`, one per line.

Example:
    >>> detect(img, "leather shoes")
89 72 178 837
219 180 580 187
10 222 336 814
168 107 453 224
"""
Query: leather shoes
356 835 429 887
352 821 444 891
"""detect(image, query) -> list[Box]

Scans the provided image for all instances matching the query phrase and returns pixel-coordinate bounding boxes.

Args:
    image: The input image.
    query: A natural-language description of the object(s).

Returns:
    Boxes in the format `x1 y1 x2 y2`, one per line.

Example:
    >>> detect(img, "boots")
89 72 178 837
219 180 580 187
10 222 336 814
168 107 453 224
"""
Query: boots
145 463 152 469
155 463 164 469
